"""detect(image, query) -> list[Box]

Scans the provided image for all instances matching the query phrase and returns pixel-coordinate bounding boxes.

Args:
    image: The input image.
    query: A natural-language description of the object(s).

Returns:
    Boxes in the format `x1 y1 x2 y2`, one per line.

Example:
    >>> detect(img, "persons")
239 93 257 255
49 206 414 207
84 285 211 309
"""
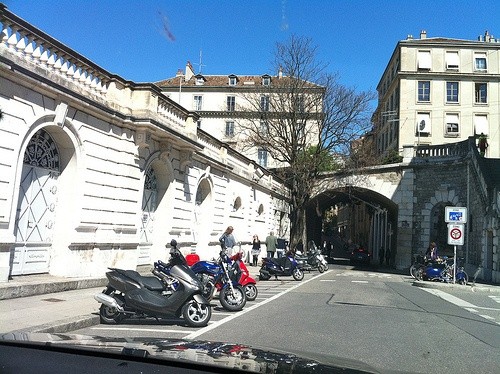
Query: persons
220 226 236 260
251 234 261 266
296 239 303 251
426 242 438 259
322 240 328 255
264 232 278 258
386 248 391 265
379 246 385 264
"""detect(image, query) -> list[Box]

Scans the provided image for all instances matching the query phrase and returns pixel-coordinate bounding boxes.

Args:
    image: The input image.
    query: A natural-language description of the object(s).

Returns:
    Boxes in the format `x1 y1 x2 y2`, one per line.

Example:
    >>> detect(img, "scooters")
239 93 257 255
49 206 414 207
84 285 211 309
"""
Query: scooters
411 253 468 286
259 246 305 281
286 240 329 274
93 238 212 327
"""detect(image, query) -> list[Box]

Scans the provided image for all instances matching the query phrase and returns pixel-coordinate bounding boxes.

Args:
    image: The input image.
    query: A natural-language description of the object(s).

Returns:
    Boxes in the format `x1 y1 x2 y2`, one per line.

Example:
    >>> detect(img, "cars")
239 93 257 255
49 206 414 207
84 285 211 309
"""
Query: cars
351 248 371 267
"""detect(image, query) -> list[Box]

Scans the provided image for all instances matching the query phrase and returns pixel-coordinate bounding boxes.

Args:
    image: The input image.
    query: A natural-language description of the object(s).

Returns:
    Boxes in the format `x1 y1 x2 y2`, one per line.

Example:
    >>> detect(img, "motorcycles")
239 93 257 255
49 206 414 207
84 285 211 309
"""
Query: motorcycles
152 240 258 312
